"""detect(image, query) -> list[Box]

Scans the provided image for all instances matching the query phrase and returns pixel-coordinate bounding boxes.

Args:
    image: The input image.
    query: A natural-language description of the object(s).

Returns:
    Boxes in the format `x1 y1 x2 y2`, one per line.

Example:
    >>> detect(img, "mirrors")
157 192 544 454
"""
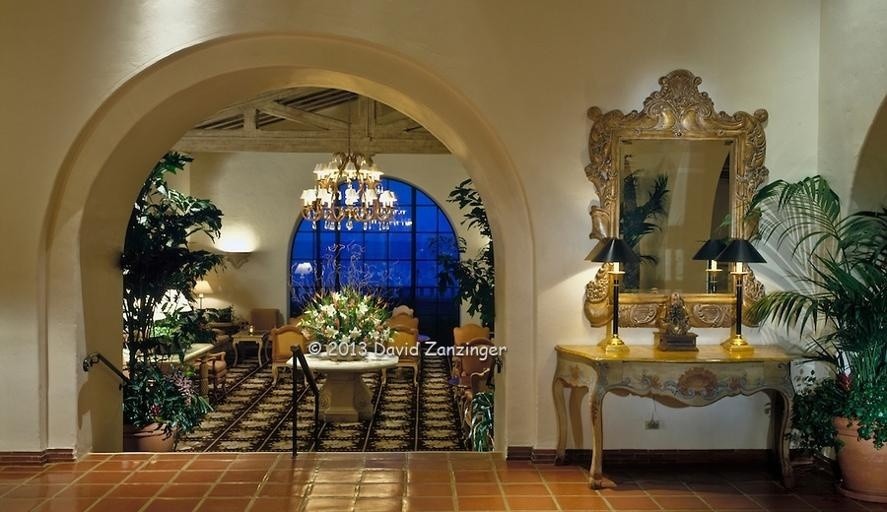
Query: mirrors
583 68 771 329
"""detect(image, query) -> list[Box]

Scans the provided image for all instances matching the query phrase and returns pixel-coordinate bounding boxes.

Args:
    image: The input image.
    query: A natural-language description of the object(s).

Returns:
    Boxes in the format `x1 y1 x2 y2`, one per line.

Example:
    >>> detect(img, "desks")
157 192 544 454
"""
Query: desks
123 342 215 408
419 334 431 343
286 350 400 422
551 344 803 490
230 329 270 368
449 377 470 389
207 322 234 327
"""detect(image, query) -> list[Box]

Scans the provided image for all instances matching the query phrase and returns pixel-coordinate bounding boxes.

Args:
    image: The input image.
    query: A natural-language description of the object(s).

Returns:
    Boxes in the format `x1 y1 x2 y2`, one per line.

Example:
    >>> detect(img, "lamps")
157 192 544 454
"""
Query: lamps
191 280 213 309
691 239 728 293
299 100 413 233
592 238 641 352
714 239 768 351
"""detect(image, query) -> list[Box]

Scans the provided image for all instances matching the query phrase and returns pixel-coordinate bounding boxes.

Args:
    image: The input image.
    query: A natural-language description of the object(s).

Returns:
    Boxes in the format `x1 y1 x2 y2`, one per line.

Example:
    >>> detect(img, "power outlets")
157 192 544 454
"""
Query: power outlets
645 420 659 429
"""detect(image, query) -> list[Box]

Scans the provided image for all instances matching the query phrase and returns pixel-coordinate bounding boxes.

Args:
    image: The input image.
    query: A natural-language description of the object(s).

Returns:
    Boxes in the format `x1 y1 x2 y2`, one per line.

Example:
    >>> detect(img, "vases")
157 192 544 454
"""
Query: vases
325 344 366 361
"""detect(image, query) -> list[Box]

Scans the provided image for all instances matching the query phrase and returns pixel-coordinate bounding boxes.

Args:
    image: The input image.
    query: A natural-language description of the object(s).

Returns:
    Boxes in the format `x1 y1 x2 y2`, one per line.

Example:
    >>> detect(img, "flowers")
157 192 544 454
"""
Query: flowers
123 364 213 452
294 279 401 345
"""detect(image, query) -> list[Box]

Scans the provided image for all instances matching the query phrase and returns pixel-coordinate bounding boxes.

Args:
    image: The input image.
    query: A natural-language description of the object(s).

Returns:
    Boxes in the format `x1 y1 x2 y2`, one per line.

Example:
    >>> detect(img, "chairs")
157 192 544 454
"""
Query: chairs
380 325 421 387
391 305 413 317
270 326 306 387
287 314 305 325
453 324 491 346
461 369 495 435
243 308 279 349
388 312 419 328
460 337 496 376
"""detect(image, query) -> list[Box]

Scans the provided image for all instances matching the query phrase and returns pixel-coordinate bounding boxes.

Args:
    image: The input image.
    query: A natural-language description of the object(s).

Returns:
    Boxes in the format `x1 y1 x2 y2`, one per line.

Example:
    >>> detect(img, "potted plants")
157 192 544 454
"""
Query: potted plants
214 307 233 322
782 339 887 504
150 318 191 363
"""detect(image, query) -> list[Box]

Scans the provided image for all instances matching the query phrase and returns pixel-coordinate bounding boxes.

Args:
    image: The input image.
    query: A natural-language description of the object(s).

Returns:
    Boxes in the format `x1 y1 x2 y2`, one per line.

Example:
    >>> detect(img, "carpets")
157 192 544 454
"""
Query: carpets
176 354 470 452
